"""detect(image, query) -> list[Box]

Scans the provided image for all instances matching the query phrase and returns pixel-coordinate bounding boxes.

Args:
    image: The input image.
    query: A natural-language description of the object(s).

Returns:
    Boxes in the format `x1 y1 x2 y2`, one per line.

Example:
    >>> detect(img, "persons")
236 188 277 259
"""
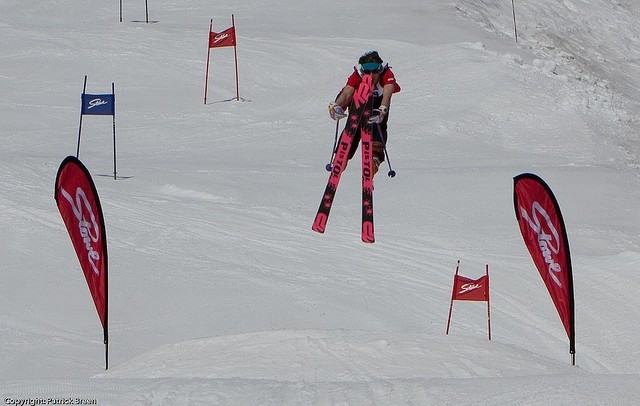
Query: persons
327 50 401 190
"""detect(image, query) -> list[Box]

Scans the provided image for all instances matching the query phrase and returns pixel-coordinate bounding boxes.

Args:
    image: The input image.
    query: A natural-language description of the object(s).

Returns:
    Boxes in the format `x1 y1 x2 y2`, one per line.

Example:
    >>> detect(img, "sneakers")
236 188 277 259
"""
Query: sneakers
372 159 379 173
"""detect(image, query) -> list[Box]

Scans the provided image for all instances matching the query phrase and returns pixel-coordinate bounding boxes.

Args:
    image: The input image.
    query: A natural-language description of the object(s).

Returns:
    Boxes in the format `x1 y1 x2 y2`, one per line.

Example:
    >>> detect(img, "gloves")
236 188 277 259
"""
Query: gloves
368 106 388 124
328 103 347 120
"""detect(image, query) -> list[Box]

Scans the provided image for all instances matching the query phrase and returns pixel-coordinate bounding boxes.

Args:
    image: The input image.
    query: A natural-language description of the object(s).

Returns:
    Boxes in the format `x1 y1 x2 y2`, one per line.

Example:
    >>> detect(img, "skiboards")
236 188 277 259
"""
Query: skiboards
311 75 374 243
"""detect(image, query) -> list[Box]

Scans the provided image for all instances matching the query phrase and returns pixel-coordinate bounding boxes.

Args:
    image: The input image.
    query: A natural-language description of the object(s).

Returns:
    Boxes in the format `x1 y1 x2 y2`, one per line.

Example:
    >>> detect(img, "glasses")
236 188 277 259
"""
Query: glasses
363 69 382 74
